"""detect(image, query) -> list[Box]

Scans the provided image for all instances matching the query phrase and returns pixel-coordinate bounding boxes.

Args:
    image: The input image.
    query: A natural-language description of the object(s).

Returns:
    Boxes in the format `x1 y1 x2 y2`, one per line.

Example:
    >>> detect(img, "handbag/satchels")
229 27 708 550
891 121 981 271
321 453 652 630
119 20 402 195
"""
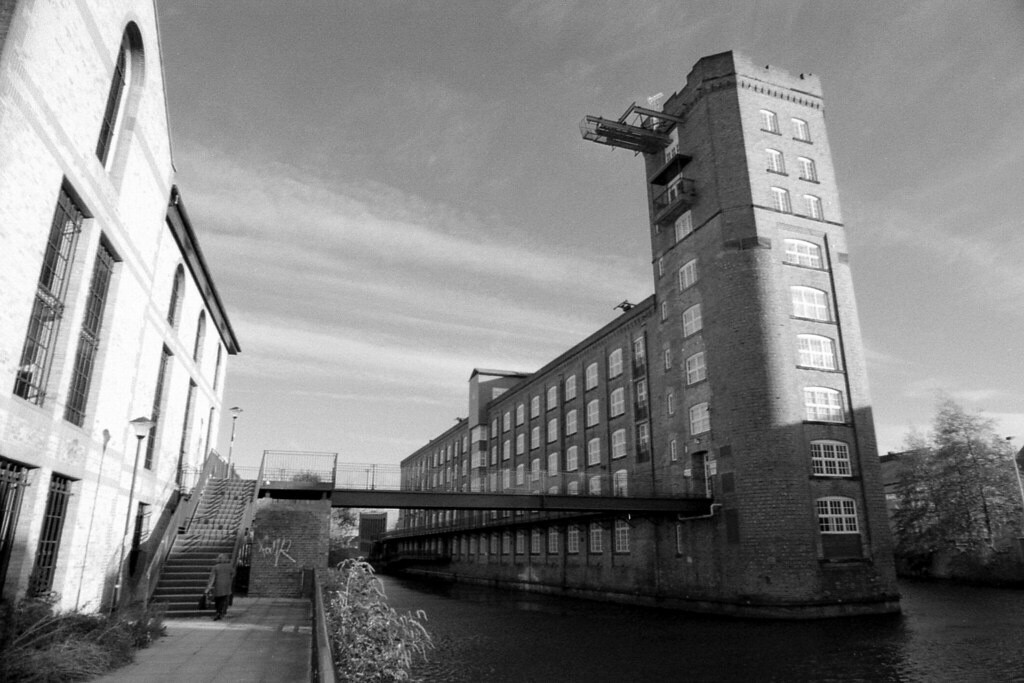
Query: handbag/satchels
198 593 211 610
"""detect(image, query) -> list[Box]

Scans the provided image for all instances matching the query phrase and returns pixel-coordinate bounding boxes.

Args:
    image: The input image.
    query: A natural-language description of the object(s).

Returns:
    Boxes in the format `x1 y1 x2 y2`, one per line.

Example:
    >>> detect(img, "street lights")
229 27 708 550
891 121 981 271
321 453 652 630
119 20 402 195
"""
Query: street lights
109 417 158 616
223 407 241 478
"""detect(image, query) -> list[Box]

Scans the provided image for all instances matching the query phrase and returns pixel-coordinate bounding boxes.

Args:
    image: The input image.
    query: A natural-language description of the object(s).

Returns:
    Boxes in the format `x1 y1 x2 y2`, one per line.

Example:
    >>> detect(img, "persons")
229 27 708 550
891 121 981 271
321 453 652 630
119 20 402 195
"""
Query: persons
204 553 236 621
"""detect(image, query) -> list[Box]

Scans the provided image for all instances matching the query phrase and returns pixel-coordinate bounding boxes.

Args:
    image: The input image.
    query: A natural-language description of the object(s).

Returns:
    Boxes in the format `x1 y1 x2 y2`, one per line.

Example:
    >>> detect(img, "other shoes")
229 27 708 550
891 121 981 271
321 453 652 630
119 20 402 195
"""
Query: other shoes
213 613 220 621
